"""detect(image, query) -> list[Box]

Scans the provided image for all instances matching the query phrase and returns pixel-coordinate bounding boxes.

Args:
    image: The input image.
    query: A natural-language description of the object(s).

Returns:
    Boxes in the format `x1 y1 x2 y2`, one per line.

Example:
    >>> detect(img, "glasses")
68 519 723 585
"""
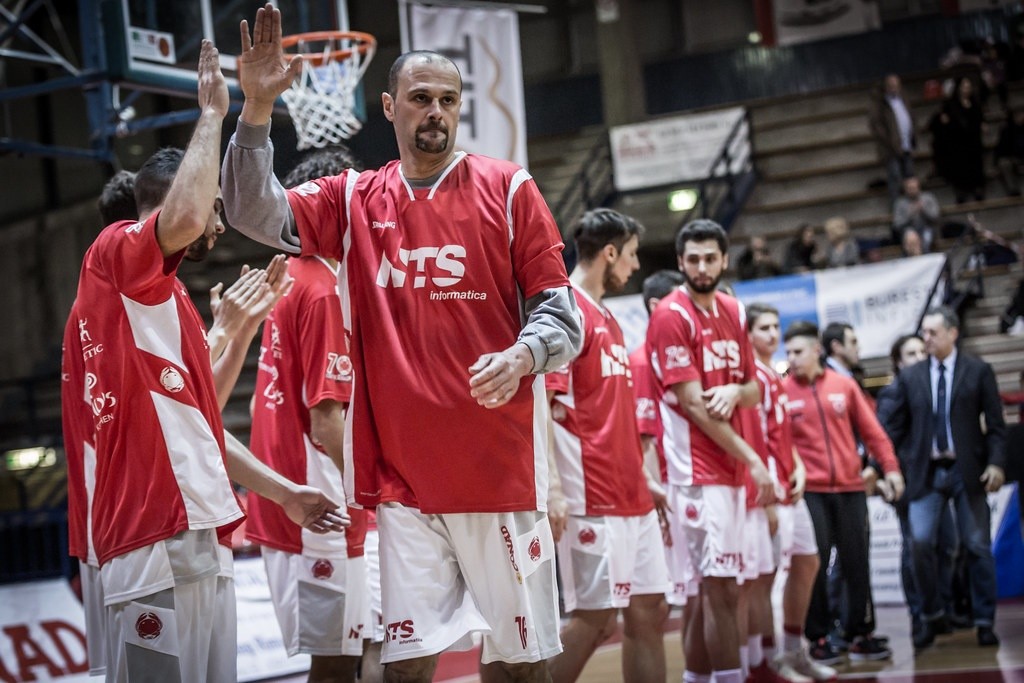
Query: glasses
918 327 937 336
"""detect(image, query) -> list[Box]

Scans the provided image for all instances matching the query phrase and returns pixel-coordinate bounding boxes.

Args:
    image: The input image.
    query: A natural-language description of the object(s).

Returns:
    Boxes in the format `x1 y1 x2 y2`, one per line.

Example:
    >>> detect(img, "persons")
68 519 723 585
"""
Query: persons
77 40 353 683
628 219 905 683
547 207 675 683
860 306 1005 647
219 4 585 683
240 150 382 683
735 34 1024 281
58 168 296 678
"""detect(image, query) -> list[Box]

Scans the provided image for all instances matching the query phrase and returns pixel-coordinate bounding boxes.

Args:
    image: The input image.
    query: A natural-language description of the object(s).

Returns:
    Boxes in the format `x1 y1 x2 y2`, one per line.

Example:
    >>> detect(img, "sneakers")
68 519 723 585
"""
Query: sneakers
849 636 891 659
745 658 792 683
977 625 998 645
913 618 954 648
768 640 837 683
810 638 846 664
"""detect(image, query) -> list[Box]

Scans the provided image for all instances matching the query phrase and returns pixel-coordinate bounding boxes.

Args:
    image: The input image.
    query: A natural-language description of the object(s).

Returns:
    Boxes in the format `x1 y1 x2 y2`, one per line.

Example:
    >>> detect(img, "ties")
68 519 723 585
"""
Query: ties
937 362 949 453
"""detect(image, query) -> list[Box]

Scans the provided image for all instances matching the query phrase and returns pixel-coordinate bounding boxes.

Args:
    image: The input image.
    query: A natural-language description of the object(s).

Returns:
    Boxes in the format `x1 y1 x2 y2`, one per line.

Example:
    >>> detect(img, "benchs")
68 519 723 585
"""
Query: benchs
218 76 1024 432
